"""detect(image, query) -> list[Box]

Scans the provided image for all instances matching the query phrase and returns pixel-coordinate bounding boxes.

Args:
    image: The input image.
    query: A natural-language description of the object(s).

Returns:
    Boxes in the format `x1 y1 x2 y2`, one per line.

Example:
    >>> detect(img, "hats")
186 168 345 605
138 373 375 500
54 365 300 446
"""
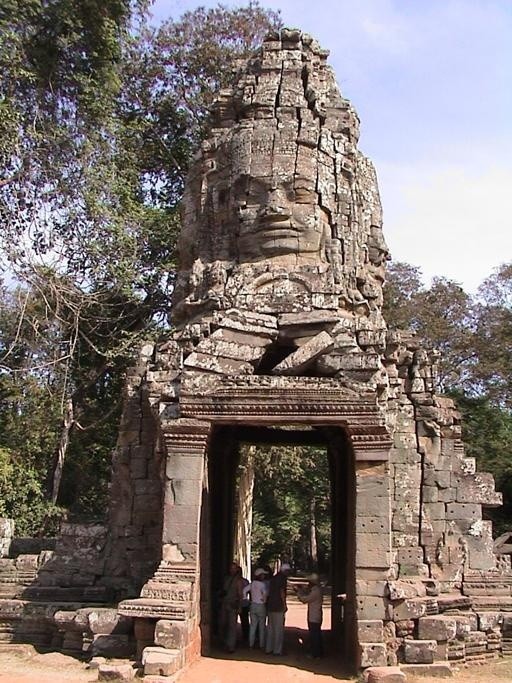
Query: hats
253 567 269 576
280 563 294 572
306 572 321 586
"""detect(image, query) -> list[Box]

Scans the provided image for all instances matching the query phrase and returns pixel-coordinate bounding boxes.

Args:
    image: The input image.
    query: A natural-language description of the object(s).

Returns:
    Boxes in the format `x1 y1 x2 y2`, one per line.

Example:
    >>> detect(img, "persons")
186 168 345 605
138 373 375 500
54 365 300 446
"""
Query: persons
218 563 291 657
293 572 323 659
